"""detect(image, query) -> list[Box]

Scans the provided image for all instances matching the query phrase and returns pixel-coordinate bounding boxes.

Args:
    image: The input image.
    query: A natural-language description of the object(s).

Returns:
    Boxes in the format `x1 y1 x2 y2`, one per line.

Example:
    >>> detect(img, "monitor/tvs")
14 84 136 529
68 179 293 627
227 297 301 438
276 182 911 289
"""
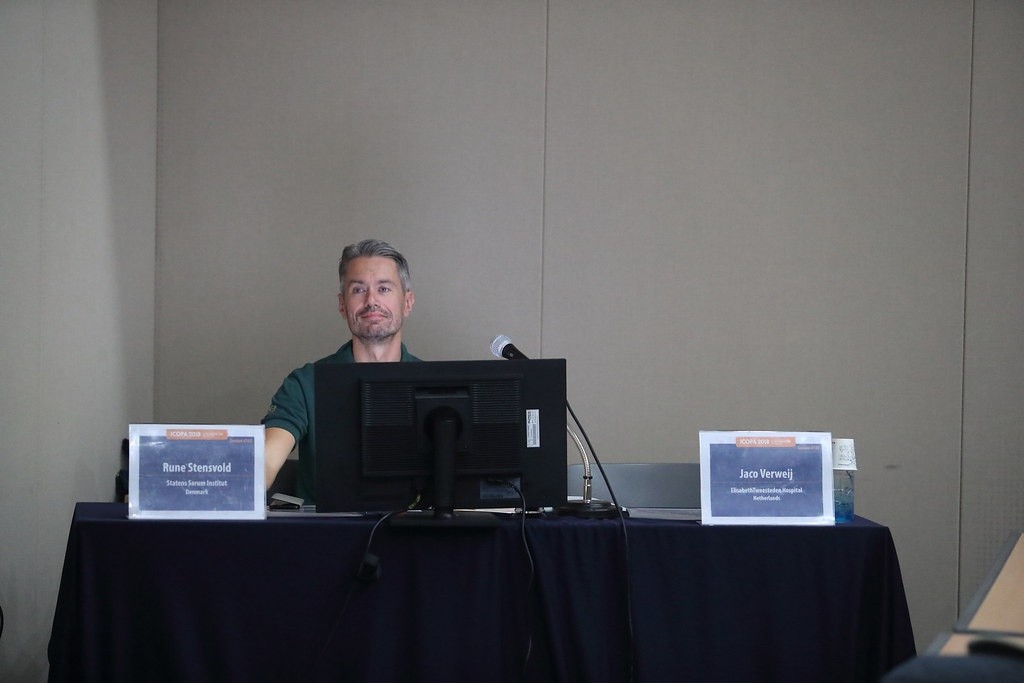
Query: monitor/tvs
313 358 567 526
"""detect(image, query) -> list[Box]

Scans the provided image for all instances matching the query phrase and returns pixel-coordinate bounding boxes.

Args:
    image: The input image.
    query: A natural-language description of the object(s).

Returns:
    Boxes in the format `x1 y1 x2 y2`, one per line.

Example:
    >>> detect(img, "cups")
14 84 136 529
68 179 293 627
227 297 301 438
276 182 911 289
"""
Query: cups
831 437 858 472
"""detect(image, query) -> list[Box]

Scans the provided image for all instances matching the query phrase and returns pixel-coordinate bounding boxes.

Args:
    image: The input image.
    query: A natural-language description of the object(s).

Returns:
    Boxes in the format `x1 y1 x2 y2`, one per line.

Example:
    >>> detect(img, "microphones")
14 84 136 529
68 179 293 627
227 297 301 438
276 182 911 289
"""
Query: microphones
489 335 530 361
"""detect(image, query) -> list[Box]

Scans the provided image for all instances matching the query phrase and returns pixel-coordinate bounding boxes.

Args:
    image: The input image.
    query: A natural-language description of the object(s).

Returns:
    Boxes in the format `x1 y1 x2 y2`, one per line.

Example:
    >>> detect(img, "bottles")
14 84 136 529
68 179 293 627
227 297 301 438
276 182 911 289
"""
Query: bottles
832 469 855 523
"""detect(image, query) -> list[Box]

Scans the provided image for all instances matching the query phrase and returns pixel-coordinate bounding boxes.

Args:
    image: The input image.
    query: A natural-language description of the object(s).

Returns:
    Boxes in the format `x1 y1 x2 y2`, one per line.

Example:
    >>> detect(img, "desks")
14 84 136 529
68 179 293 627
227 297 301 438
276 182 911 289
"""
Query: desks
951 531 1024 635
937 631 1024 656
45 501 916 683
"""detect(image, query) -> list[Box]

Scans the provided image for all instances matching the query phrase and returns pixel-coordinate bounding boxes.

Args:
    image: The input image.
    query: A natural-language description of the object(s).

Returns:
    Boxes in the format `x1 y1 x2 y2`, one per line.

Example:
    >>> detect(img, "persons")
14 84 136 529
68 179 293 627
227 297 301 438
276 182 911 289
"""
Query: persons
260 239 425 491
